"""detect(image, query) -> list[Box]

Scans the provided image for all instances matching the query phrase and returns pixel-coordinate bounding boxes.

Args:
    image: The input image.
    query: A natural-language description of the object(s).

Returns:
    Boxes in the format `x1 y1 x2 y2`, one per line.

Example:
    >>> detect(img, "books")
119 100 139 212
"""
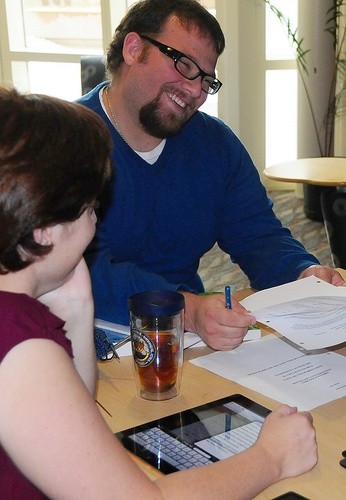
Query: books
95 292 261 359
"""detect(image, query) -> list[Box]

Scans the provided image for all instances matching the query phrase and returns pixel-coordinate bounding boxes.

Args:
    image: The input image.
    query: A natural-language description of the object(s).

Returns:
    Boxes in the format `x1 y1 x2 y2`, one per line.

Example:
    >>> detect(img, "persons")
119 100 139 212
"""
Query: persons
0 85 319 500
69 0 346 352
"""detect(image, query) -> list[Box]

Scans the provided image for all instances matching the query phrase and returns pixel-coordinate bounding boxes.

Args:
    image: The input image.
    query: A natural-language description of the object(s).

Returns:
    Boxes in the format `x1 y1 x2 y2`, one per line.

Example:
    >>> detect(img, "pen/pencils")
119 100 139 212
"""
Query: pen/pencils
224 284 231 309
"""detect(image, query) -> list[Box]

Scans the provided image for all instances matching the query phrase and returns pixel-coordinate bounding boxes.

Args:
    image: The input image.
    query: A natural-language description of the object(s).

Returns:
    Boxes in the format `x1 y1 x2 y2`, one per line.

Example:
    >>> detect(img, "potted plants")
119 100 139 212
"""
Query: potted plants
264 0 346 222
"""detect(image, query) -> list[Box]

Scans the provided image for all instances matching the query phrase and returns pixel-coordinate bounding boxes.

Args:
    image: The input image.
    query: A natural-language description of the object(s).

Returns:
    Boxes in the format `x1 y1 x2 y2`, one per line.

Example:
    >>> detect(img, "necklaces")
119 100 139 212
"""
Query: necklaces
105 85 128 145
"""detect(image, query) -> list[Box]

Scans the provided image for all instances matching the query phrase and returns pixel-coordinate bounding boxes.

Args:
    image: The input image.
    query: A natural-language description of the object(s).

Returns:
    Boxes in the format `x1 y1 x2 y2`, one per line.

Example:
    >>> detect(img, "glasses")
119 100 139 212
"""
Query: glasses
140 33 223 95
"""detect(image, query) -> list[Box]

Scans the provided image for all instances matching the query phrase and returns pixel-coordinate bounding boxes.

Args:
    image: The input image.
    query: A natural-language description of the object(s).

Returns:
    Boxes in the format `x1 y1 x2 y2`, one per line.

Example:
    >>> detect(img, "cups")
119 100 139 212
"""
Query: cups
129 288 186 401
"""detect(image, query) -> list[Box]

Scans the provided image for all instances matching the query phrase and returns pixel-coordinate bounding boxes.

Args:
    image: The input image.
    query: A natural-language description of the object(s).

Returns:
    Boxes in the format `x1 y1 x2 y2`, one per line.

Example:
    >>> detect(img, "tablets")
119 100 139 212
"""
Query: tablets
113 394 273 476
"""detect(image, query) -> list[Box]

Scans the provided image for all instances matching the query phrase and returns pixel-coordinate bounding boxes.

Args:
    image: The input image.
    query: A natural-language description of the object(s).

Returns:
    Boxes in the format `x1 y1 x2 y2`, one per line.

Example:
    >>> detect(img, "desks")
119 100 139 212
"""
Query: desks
262 156 346 271
93 269 346 500
